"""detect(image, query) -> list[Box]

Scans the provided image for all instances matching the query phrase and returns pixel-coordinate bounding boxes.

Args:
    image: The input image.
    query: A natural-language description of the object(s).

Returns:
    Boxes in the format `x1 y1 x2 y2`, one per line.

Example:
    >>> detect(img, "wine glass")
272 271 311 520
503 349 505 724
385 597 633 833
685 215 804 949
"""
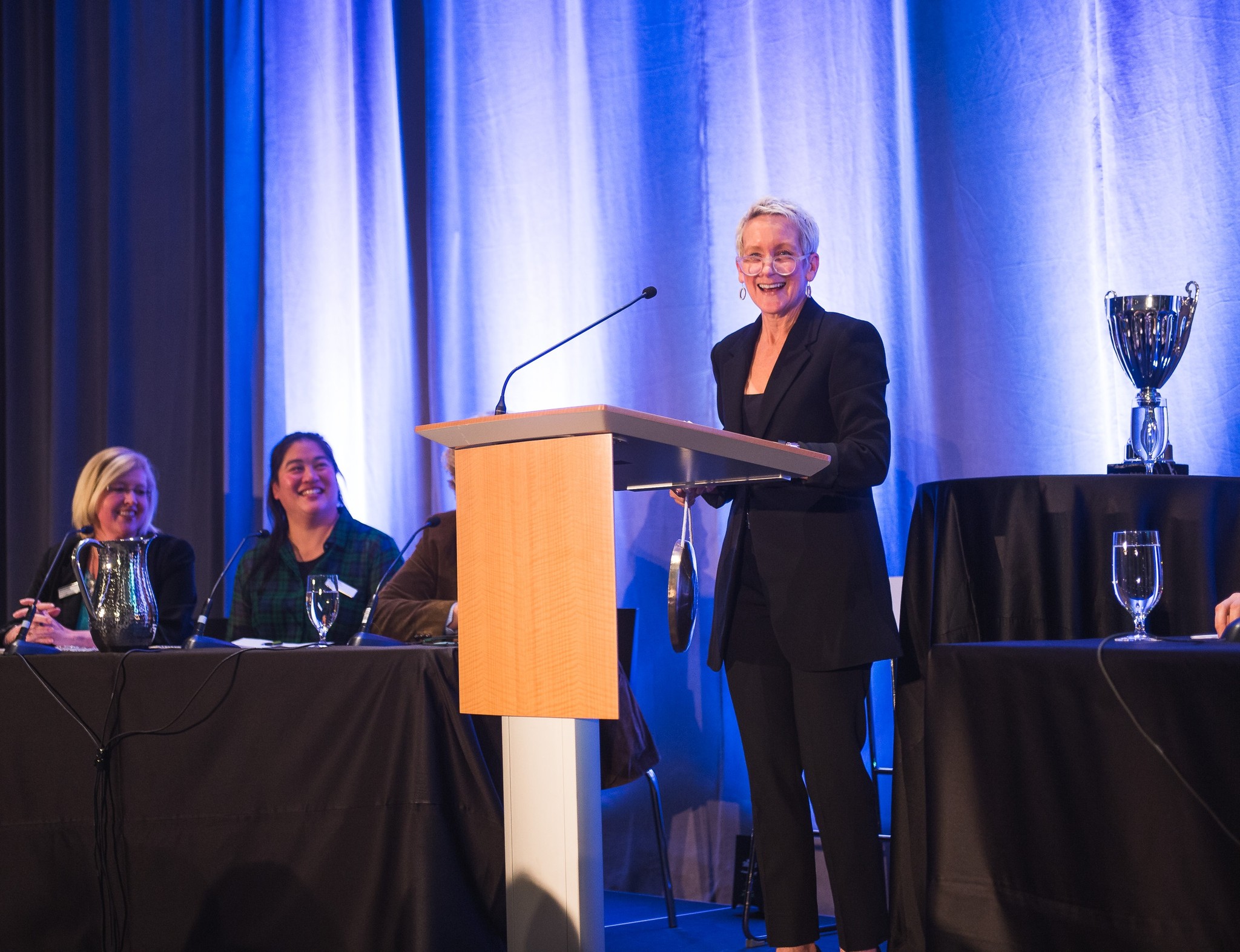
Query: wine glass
1113 530 1165 641
305 574 340 647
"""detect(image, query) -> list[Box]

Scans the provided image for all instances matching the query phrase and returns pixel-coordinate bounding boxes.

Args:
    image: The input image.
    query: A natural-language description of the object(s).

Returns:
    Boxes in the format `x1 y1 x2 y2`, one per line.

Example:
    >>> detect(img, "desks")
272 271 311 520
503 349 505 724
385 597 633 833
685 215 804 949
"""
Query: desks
887 474 1240 952
0 645 512 952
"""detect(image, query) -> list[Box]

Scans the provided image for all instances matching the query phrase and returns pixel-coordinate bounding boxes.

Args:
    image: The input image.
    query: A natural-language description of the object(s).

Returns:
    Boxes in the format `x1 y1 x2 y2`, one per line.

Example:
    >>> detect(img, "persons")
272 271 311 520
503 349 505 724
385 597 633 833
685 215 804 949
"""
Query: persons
1214 593 1240 639
368 447 459 644
668 198 899 952
230 431 405 644
0 447 197 649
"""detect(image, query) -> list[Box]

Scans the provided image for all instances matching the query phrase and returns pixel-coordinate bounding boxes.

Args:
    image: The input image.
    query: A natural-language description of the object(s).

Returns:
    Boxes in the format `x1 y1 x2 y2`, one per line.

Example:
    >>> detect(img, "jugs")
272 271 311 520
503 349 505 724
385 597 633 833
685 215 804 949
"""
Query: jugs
71 532 159 653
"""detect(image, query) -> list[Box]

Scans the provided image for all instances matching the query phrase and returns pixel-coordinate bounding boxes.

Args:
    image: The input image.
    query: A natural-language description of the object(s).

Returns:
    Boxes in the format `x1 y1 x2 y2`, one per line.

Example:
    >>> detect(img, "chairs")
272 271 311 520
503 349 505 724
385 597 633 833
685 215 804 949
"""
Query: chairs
599 608 685 929
742 576 904 941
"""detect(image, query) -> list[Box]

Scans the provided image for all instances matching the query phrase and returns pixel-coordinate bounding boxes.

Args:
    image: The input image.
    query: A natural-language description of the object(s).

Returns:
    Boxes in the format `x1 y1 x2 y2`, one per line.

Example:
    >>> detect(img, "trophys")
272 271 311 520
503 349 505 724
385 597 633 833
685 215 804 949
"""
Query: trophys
1104 281 1200 475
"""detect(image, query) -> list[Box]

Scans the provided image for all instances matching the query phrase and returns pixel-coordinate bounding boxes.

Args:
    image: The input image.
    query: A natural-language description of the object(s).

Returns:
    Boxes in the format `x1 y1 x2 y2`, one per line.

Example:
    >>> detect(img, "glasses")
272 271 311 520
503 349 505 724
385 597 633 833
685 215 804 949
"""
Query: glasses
737 253 812 276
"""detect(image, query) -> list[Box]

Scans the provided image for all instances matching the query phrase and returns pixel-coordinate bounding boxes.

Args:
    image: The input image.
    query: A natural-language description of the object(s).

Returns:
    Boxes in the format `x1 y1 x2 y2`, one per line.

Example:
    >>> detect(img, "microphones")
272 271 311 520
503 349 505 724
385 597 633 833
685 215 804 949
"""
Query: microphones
344 516 441 646
180 529 270 649
3 525 93 655
493 286 657 416
